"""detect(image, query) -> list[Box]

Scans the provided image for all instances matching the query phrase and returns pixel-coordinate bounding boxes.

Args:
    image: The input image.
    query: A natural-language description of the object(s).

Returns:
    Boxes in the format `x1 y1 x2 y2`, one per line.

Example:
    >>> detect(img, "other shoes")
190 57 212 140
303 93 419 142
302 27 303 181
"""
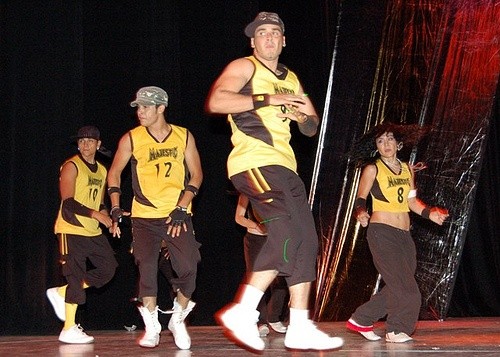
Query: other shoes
259 326 269 338
268 321 287 333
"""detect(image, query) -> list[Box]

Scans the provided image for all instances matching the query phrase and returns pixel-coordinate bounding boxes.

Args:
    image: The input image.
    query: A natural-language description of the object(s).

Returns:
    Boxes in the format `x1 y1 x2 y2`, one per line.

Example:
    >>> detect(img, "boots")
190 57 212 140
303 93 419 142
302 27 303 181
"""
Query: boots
167 297 196 350
138 305 162 348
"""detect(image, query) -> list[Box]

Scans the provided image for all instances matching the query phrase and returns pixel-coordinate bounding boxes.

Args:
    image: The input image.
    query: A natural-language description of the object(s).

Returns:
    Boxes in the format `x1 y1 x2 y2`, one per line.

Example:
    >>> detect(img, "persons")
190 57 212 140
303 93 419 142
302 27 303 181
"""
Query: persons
234 192 287 336
47 125 118 344
205 12 345 352
341 121 449 343
107 86 203 349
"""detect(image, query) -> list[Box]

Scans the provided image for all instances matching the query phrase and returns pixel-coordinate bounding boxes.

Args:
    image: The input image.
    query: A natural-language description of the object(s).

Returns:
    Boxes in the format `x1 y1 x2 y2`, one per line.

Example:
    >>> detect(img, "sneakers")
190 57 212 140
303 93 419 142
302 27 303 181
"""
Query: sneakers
347 318 382 340
213 303 264 351
385 332 413 343
45 287 66 321
58 325 94 345
283 323 343 351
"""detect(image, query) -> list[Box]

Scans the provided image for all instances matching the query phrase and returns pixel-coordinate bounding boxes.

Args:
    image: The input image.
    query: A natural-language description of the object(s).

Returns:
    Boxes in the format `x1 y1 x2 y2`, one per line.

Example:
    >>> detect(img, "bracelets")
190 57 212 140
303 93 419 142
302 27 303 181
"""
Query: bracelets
108 187 121 195
184 184 198 195
422 205 433 219
353 207 367 218
253 93 268 109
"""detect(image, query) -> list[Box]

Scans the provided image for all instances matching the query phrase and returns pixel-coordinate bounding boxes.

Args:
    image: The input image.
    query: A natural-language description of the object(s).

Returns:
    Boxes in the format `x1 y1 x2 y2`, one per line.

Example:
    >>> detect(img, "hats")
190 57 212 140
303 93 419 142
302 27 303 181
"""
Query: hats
129 86 169 107
70 125 100 141
245 11 285 37
